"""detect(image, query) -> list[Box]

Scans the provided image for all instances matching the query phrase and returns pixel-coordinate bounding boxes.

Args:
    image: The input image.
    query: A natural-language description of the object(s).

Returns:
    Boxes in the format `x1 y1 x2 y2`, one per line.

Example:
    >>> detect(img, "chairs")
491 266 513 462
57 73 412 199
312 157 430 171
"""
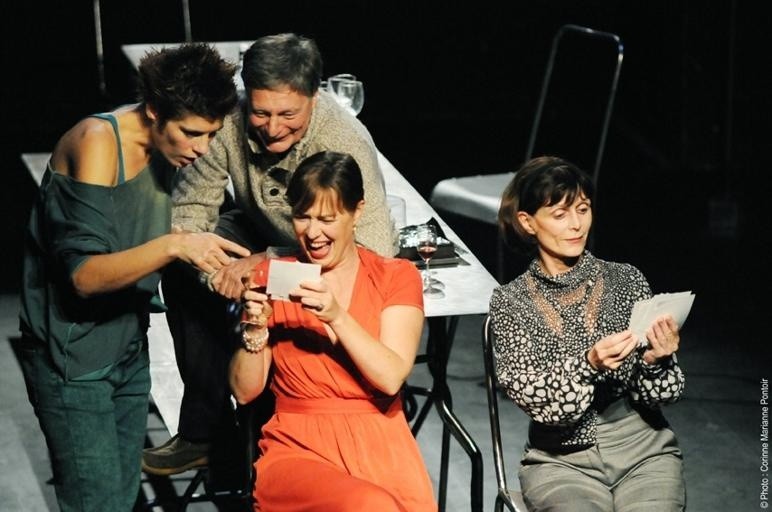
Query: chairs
482 315 525 512
227 350 417 510
429 24 622 232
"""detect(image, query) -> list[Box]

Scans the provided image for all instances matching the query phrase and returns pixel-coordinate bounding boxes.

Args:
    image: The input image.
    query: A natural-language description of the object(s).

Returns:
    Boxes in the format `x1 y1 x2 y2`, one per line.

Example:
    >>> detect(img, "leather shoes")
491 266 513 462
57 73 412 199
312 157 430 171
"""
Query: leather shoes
143 431 214 473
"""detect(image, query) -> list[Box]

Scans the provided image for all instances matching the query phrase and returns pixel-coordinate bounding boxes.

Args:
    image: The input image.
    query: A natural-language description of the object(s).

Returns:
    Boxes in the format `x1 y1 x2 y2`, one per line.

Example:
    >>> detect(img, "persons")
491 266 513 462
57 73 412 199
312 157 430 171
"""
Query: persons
141 34 401 476
19 43 250 512
489 156 686 512
229 151 438 512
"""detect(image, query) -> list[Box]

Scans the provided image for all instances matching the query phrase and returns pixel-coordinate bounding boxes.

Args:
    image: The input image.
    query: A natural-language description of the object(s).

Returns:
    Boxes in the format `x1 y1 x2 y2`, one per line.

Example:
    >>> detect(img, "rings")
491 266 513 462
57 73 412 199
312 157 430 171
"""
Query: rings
318 301 324 311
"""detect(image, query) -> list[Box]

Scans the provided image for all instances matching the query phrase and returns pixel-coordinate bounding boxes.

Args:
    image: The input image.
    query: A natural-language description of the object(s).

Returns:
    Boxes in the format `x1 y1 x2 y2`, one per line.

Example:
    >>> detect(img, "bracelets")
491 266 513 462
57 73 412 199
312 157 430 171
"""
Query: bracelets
241 329 270 353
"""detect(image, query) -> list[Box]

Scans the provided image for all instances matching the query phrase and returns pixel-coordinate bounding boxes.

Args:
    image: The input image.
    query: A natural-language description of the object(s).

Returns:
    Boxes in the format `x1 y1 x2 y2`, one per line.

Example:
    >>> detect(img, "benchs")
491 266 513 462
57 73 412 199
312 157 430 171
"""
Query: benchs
18 151 242 511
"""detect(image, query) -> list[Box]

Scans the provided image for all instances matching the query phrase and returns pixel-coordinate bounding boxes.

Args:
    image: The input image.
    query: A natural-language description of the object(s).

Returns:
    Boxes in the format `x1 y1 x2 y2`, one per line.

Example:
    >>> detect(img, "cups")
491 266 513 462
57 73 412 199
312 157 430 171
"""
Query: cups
418 232 438 295
417 225 438 285
338 81 365 117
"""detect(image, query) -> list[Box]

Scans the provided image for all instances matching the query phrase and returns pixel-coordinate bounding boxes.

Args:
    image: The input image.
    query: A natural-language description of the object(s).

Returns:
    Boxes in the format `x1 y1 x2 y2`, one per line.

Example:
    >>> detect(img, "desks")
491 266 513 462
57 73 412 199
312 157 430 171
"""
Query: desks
122 42 503 512
